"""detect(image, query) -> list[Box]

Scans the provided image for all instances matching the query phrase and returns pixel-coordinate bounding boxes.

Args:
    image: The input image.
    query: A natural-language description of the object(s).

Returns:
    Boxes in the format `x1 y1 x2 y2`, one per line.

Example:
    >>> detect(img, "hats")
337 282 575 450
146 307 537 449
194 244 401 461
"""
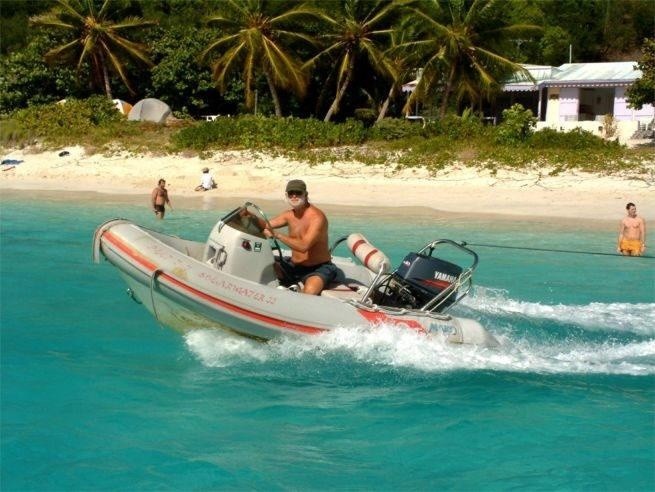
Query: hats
285 178 307 193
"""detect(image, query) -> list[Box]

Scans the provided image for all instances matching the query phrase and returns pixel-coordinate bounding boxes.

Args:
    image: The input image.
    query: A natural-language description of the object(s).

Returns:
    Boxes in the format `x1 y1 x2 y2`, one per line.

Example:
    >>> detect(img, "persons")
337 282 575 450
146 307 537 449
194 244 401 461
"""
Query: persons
195 167 214 191
152 179 169 219
616 203 646 257
239 180 337 295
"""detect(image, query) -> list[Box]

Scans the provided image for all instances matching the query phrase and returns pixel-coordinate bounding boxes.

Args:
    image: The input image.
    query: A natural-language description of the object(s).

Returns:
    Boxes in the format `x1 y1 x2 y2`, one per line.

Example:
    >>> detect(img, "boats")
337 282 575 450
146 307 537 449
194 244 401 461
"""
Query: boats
92 202 502 364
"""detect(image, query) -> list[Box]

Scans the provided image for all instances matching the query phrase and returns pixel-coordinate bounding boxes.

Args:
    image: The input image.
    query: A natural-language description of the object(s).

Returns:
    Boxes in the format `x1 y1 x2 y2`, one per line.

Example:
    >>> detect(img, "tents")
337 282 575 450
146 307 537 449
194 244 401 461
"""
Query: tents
112 98 133 116
127 98 174 123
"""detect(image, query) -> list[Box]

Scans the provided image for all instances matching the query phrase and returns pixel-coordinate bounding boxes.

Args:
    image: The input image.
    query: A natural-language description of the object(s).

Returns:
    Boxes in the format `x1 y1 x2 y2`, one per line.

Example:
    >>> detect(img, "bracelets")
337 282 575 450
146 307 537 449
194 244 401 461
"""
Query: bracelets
642 244 646 247
